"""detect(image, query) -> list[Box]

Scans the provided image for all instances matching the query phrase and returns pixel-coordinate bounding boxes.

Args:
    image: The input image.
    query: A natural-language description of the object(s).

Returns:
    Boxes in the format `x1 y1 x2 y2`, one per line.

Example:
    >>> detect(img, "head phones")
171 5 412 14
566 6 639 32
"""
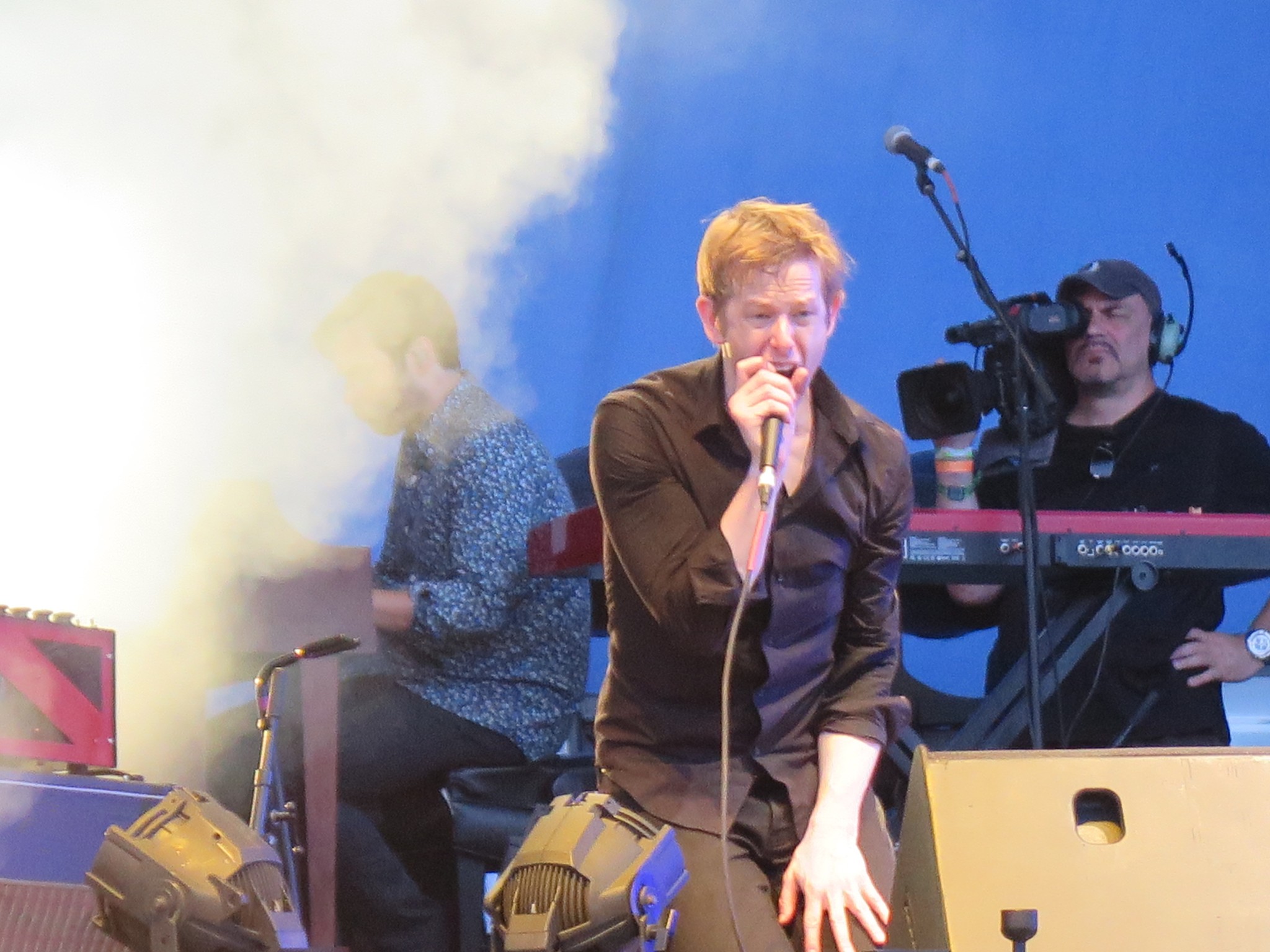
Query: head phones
1157 323 1182 362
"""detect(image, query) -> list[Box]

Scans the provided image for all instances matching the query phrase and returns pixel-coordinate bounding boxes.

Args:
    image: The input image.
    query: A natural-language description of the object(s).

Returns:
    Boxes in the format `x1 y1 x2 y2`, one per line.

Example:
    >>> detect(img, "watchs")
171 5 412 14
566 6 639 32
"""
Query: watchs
1245 626 1270 666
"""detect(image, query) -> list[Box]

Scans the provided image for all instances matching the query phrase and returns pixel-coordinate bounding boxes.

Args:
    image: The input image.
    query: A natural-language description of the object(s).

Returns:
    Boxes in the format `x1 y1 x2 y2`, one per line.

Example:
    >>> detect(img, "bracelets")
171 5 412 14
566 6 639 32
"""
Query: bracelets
927 445 982 500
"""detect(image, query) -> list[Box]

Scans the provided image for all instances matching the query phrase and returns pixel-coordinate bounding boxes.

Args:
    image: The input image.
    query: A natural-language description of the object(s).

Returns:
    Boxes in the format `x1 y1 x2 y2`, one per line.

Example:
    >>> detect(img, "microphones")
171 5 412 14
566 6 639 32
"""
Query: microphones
885 125 944 174
1166 239 1194 355
757 415 782 503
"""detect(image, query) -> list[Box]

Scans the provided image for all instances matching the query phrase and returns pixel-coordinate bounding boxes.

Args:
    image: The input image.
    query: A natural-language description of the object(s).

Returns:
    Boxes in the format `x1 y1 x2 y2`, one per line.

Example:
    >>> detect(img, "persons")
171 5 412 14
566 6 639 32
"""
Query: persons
588 199 913 952
925 260 1270 750
213 273 592 952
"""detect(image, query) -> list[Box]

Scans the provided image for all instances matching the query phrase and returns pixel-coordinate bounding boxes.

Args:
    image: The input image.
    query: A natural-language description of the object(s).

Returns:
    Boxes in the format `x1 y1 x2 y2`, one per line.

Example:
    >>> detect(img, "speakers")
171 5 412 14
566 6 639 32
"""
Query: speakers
884 743 1269 950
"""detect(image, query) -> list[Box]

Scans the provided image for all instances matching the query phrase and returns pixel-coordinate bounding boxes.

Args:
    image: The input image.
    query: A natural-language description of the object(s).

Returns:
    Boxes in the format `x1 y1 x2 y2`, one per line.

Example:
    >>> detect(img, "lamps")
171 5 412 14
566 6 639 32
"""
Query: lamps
481 789 691 952
84 786 310 952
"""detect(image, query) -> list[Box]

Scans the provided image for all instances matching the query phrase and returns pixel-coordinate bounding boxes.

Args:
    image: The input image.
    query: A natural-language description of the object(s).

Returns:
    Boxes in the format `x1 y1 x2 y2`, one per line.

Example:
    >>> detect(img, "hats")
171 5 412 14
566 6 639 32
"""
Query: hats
1054 258 1163 317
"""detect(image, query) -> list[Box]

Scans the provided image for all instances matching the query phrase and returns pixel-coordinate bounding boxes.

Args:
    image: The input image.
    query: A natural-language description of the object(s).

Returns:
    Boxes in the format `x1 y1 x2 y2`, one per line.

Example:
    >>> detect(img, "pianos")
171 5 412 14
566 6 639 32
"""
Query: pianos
528 494 1269 792
